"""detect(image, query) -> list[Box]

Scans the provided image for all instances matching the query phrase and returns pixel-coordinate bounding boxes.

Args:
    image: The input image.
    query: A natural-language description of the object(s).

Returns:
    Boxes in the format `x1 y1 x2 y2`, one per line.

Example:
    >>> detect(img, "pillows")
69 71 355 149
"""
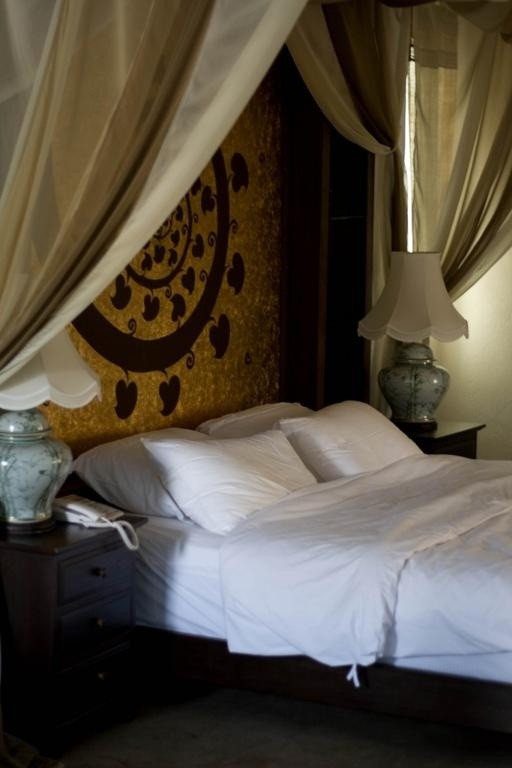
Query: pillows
194 399 317 437
64 423 197 522
273 397 424 482
142 431 320 535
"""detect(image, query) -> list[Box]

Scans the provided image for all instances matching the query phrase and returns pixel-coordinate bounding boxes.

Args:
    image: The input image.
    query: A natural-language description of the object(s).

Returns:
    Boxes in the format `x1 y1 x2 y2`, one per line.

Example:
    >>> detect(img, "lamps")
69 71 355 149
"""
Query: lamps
352 248 472 427
0 326 101 528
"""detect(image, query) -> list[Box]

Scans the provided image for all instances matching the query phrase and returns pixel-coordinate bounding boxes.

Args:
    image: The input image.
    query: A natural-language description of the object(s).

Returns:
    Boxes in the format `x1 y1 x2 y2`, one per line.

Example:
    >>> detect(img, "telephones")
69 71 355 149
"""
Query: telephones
54 494 124 525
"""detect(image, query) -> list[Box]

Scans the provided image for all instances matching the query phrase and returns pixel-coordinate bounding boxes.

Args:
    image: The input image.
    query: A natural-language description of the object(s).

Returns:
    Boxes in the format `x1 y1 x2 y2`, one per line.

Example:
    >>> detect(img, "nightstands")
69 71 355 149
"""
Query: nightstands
1 502 148 766
393 420 484 458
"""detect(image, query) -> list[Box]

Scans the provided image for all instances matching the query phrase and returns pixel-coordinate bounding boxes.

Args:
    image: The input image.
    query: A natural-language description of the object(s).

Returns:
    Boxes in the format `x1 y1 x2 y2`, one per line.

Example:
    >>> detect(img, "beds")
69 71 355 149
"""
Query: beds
85 454 511 686
